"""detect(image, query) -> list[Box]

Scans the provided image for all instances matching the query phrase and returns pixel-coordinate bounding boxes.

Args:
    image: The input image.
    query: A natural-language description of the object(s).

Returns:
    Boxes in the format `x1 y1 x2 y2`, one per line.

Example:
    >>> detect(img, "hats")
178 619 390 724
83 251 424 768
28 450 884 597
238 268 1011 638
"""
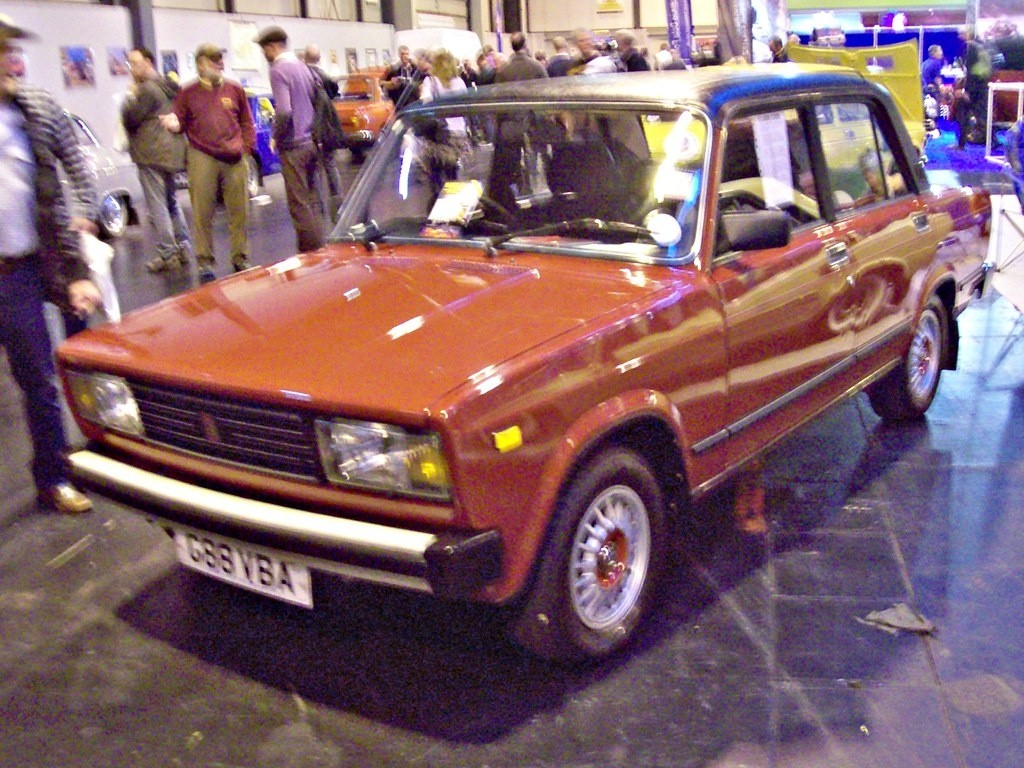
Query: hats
195 43 226 59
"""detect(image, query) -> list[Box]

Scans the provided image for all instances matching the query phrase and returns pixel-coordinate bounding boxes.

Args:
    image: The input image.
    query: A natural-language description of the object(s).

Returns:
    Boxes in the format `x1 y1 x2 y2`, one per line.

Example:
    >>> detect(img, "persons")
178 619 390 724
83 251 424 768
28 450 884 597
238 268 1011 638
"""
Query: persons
922 23 1000 150
158 44 258 286
257 24 349 253
0 20 104 512
770 34 801 63
120 44 186 274
864 167 883 196
377 31 689 218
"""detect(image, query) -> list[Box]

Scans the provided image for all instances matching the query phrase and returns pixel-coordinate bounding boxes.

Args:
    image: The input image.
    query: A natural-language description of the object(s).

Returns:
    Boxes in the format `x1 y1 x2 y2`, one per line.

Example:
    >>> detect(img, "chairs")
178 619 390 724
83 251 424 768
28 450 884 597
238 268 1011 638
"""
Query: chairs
535 139 645 227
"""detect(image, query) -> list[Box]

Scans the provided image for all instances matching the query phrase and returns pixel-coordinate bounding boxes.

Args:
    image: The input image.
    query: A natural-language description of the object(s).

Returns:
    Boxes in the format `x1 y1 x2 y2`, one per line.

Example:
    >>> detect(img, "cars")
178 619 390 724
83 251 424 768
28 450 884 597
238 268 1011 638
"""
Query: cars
327 64 396 152
66 112 150 241
50 62 996 673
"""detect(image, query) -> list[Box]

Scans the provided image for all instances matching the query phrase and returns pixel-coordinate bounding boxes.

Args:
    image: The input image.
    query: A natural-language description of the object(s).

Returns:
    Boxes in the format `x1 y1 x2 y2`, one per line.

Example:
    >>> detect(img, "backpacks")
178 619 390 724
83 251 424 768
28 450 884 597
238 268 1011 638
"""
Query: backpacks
306 63 344 151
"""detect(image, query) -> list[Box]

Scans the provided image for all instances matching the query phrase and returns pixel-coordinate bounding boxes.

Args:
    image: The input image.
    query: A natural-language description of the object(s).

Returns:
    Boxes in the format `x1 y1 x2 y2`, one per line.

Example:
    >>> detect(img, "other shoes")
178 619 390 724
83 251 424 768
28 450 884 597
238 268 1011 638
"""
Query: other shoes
179 249 188 262
38 483 92 512
199 264 216 283
233 261 252 272
147 254 180 270
